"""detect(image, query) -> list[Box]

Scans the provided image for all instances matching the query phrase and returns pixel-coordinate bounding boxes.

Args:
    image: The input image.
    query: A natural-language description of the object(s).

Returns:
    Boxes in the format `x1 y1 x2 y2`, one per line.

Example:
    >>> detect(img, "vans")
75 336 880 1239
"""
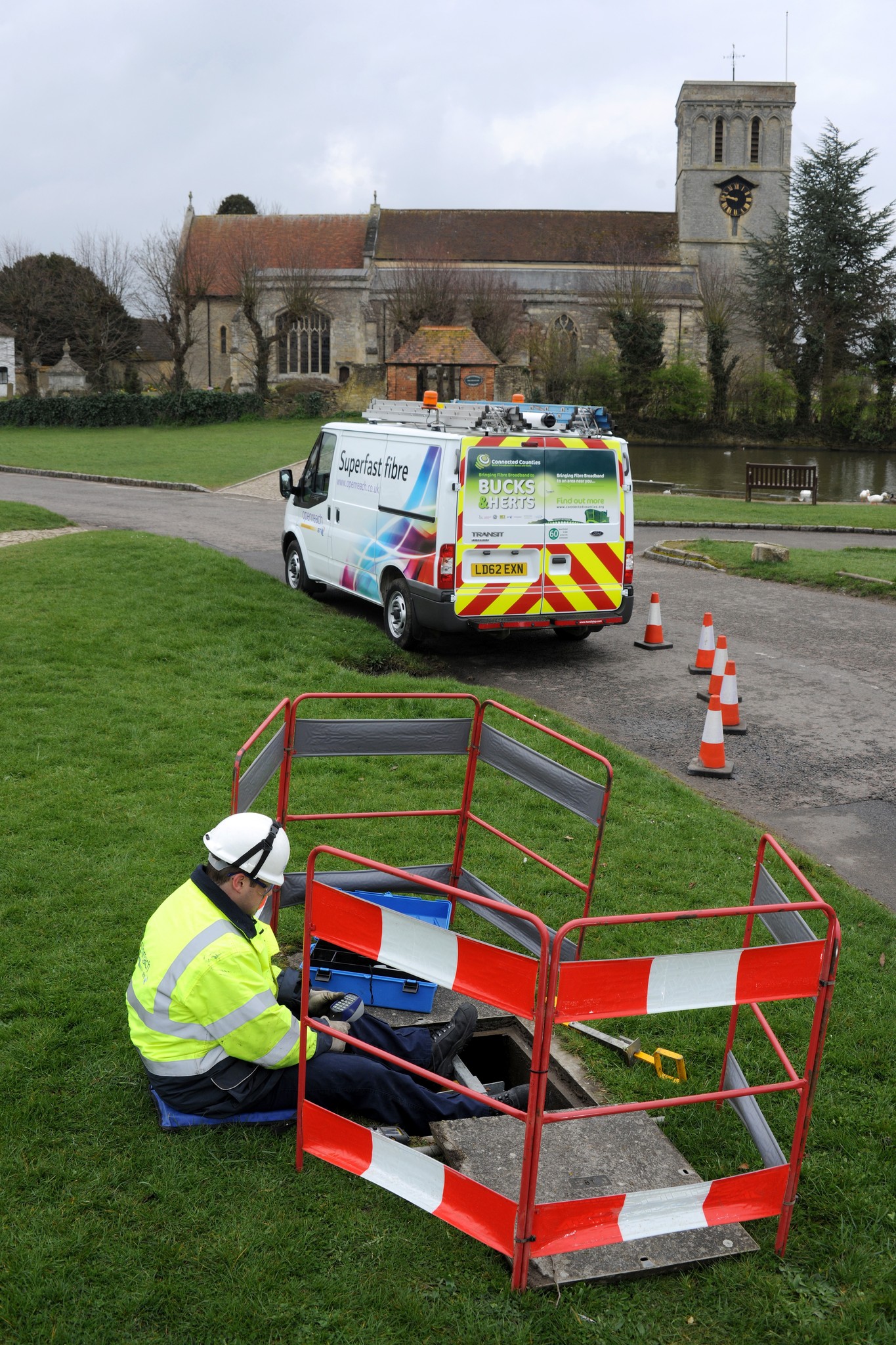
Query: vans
279 389 635 651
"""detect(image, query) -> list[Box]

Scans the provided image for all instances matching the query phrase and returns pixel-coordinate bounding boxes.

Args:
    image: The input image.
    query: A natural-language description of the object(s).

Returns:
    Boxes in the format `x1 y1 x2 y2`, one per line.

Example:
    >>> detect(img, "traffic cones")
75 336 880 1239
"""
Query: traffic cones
688 693 734 778
717 659 749 735
688 612 717 674
697 634 743 704
633 591 675 652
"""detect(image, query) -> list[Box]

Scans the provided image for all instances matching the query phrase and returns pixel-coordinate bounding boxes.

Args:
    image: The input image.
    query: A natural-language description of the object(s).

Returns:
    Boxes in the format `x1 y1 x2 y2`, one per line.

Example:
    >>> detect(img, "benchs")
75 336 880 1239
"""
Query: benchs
746 462 818 505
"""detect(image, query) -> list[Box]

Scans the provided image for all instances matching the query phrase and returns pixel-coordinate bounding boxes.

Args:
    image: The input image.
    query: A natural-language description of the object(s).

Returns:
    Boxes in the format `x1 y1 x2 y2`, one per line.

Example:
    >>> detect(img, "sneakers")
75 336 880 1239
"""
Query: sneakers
488 1084 529 1117
418 1003 478 1091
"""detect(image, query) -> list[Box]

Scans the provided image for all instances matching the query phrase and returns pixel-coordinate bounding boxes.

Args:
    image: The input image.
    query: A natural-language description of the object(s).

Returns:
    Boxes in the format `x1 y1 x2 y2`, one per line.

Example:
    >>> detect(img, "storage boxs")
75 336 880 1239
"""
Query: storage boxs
299 887 453 1014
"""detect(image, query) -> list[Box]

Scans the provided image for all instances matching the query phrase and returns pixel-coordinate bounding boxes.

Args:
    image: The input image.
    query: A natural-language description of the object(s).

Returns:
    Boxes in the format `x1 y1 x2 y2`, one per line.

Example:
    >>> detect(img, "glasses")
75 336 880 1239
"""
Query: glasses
228 871 275 895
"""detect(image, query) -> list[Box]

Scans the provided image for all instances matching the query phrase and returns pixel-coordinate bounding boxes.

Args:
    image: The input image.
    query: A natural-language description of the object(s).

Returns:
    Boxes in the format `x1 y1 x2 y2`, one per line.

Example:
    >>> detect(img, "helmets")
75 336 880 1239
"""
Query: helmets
203 813 290 886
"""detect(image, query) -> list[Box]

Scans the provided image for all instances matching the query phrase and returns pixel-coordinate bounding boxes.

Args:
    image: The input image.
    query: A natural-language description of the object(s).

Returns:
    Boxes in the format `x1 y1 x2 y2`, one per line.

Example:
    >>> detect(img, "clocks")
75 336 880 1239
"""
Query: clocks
719 182 753 217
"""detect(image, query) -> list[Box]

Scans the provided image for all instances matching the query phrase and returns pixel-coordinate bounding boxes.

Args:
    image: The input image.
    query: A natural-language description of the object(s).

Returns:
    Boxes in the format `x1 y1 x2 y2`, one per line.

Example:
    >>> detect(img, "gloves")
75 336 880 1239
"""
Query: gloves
308 989 346 1017
320 1016 351 1053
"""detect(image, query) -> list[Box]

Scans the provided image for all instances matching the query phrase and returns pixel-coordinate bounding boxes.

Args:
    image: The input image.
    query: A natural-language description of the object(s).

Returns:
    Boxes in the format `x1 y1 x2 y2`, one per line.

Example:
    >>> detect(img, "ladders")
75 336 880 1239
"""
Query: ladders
362 398 618 438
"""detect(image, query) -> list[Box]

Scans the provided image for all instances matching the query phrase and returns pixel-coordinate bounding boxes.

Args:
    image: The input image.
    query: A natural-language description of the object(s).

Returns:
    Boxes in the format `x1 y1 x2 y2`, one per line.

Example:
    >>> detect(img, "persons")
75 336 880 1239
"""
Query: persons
124 813 530 1136
